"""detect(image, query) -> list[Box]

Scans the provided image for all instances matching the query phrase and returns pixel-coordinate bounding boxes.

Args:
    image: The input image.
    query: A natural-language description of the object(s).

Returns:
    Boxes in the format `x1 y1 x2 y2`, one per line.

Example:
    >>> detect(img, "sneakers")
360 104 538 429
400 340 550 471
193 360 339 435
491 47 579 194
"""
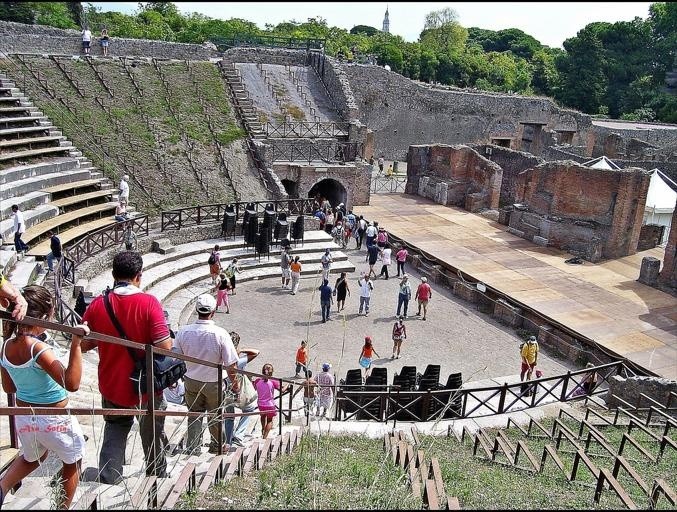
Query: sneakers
11 480 22 496
215 307 218 311
232 293 236 295
225 311 229 313
208 444 230 454
346 242 348 245
359 245 362 248
356 246 359 249
279 272 428 323
343 246 346 249
232 438 245 447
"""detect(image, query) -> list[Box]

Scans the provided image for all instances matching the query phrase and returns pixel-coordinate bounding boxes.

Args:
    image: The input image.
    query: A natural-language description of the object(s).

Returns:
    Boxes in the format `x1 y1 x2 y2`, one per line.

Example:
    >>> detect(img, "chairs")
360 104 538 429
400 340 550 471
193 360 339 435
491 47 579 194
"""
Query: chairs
221 198 308 264
332 364 463 421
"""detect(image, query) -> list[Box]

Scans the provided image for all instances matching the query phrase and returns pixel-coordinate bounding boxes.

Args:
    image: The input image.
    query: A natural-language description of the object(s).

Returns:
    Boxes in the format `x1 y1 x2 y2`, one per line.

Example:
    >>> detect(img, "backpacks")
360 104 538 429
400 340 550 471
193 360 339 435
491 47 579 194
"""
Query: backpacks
226 264 235 278
208 251 220 265
394 324 404 340
519 342 537 359
336 227 342 234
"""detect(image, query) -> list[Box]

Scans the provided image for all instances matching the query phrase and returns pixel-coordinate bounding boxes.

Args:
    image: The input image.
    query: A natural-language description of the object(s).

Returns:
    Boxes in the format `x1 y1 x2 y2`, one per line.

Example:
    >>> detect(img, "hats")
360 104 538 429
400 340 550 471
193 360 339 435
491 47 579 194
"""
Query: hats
536 371 543 376
530 336 536 343
196 293 216 313
322 363 330 369
421 277 427 282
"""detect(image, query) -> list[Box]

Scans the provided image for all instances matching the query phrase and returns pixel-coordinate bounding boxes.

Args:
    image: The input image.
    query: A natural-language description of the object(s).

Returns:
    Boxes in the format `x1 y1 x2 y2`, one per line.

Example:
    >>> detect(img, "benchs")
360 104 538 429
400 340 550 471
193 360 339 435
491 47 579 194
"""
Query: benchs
1 76 128 283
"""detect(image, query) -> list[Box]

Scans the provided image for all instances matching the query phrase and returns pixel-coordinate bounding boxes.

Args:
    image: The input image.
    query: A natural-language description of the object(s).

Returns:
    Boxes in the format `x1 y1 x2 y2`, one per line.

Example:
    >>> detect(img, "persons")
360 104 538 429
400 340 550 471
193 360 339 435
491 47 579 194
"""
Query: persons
385 164 393 179
115 201 133 236
0 284 92 511
518 336 540 383
224 257 247 296
254 364 291 440
222 331 261 453
314 363 335 419
165 378 188 407
173 293 240 459
572 363 599 397
391 319 408 360
101 28 111 57
209 245 222 287
279 247 303 295
359 337 381 380
118 175 131 209
0 272 29 323
11 205 31 254
308 191 432 324
369 156 375 167
82 26 93 56
376 155 385 175
44 229 70 278
295 340 310 380
79 252 175 484
214 273 231 315
292 370 324 417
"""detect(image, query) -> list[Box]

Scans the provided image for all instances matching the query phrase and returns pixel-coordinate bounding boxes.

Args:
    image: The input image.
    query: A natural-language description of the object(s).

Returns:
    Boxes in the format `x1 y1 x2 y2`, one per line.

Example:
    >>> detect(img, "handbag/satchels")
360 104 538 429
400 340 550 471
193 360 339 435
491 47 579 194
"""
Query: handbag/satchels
239 374 257 407
360 358 371 367
132 339 186 395
407 293 410 300
